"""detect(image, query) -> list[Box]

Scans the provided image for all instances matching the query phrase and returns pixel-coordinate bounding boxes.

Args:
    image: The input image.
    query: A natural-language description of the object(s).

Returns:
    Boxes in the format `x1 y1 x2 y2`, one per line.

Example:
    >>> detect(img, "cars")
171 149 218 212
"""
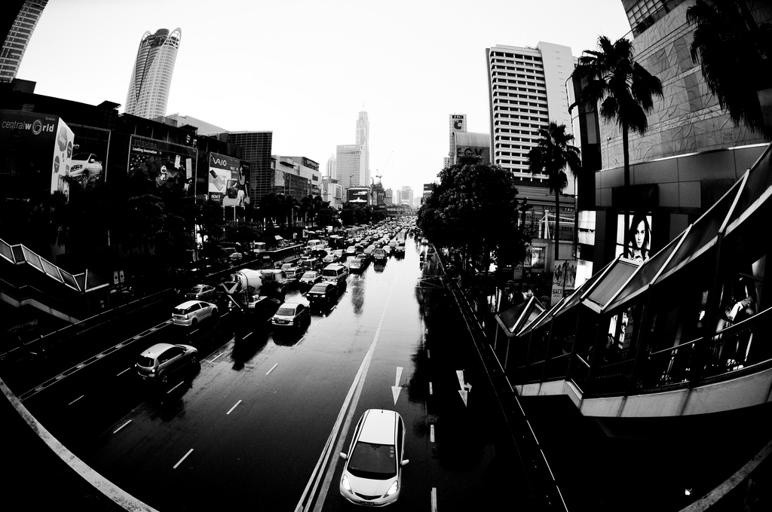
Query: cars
337 407 410 510
67 151 103 182
169 214 419 330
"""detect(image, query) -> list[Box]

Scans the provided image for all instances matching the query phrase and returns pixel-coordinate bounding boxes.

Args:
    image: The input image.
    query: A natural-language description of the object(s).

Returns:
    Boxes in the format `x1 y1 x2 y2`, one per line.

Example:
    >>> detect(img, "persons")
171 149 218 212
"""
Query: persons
620 214 651 259
552 258 579 285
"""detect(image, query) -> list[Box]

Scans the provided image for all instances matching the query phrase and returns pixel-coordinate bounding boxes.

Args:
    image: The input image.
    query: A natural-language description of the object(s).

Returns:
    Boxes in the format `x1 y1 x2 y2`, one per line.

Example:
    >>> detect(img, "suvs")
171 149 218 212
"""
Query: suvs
135 342 198 385
